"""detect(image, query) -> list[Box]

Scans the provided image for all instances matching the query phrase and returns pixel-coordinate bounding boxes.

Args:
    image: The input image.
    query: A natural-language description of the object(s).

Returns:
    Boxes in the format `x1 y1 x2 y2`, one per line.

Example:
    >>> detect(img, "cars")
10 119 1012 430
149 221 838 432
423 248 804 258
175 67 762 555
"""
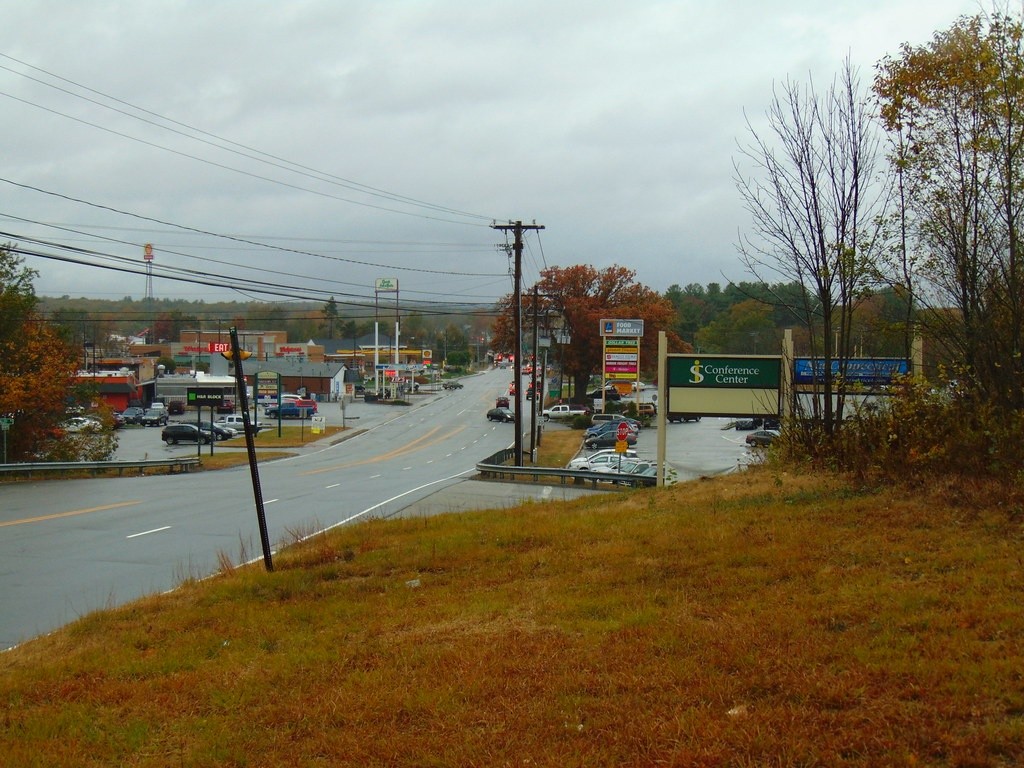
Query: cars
57 399 185 434
667 414 701 423
378 388 390 399
510 381 516 395
567 449 676 486
355 385 365 391
496 396 510 409
745 430 782 447
442 381 463 390
525 381 541 400
487 408 515 423
631 382 646 393
736 417 781 431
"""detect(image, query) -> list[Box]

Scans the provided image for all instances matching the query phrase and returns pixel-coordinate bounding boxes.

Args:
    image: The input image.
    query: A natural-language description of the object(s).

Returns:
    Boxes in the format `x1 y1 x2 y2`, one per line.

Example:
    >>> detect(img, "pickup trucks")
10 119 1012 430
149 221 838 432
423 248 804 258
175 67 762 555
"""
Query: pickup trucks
538 405 586 422
213 416 262 433
264 390 319 418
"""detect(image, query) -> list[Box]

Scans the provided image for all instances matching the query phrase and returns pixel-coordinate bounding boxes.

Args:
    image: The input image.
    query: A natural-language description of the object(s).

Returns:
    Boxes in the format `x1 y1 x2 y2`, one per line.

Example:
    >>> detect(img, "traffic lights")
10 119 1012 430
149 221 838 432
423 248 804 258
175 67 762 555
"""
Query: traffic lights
498 353 502 361
508 354 513 362
494 353 498 365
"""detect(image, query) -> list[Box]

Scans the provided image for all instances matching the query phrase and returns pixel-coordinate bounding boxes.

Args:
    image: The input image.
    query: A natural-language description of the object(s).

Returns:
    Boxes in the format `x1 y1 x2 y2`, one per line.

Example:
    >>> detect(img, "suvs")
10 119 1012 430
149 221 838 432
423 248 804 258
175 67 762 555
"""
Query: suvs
583 417 642 450
162 419 237 445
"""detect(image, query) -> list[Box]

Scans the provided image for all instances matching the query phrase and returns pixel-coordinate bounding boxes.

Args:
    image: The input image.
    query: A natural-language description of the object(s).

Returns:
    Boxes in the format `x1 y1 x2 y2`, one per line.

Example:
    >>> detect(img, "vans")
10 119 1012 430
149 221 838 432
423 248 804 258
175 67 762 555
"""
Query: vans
590 413 630 427
622 405 655 420
591 380 630 400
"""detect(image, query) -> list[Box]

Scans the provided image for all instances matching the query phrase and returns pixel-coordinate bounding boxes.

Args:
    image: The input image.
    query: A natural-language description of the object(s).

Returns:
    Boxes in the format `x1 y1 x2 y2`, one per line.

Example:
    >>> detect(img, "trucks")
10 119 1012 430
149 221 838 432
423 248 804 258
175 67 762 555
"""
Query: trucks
400 379 419 390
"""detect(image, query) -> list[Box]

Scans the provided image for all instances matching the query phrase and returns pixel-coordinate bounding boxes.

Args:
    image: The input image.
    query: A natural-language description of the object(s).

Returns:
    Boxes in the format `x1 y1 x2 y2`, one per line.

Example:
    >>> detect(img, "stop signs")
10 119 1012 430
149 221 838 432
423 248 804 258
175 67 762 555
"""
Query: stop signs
616 422 629 441
